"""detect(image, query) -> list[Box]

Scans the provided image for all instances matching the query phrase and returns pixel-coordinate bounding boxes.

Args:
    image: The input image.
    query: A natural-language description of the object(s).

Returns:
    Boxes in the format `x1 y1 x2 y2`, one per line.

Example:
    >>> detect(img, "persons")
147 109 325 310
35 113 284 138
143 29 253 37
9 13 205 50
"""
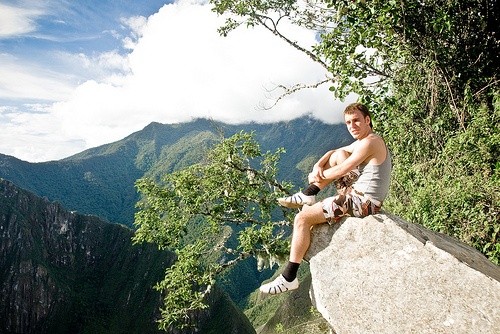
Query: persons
258 103 392 294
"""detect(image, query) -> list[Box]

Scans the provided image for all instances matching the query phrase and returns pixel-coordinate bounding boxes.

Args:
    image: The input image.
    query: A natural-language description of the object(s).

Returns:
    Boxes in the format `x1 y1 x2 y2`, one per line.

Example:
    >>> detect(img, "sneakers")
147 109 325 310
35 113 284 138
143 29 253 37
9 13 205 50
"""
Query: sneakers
278 191 315 208
260 274 298 294
